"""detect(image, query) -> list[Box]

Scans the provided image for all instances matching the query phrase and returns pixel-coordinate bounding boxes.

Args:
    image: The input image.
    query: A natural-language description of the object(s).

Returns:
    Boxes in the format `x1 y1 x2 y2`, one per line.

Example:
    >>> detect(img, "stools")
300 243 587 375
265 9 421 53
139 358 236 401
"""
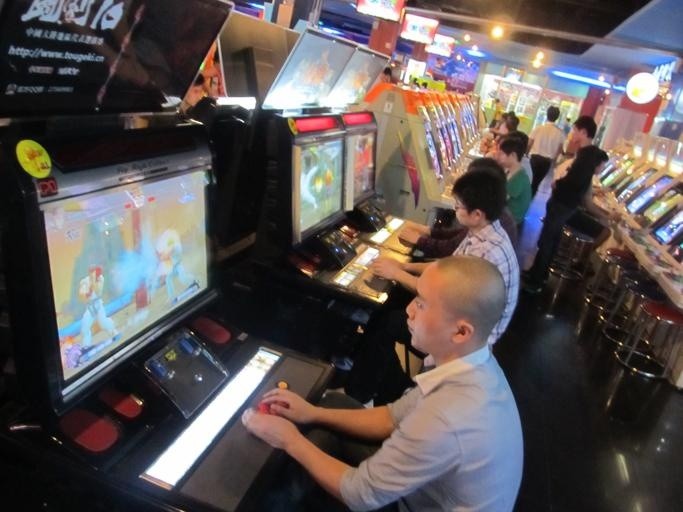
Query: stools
543 208 682 379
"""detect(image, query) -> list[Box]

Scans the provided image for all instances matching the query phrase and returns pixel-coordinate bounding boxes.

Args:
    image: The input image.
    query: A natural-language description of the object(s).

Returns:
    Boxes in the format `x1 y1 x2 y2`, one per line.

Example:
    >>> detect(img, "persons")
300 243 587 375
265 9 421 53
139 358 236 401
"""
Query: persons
383 65 393 83
177 72 207 115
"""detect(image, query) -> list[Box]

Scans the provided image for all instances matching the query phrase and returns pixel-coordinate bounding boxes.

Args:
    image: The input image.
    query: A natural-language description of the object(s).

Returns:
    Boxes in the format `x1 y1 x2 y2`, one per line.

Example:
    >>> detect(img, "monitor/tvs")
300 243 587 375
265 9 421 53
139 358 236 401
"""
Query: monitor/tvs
292 133 346 234
595 147 683 247
31 149 221 409
348 125 378 207
414 97 483 184
